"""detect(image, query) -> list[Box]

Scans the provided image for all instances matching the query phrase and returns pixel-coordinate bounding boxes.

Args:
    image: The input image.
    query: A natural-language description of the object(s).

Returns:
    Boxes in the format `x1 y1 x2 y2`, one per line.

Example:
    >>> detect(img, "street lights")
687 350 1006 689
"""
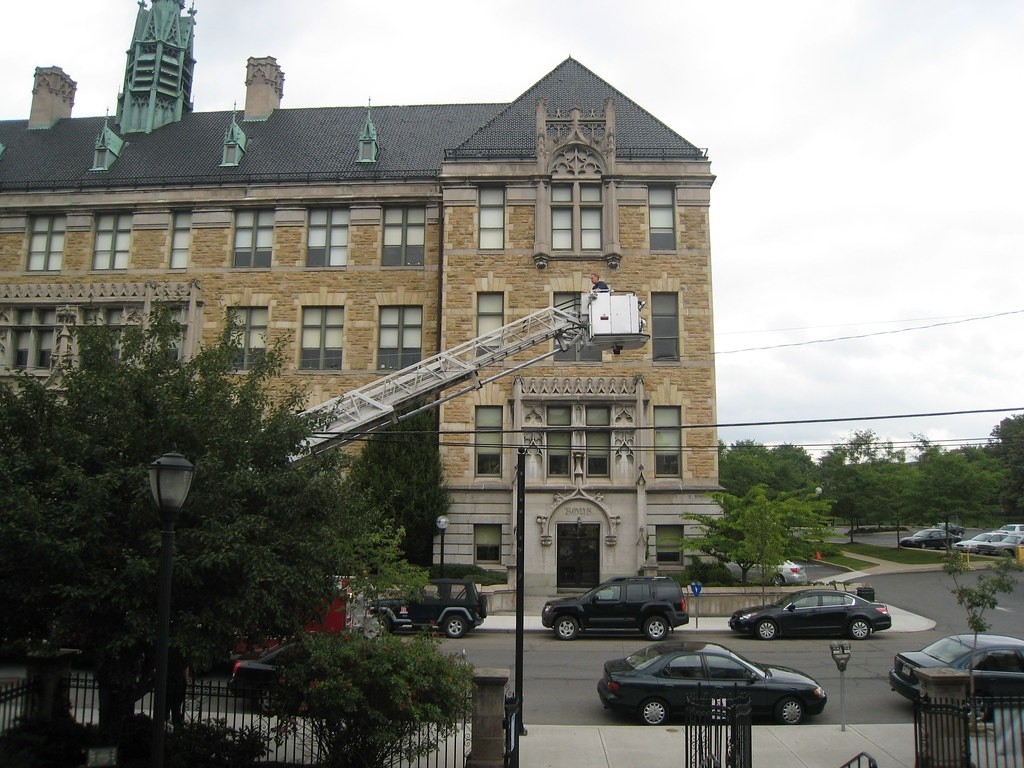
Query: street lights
815 486 825 543
144 451 196 768
436 515 449 580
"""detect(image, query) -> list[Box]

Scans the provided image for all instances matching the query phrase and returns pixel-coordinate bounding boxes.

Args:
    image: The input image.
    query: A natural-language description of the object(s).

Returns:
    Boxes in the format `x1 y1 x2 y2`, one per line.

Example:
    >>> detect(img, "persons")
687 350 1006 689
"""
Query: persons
591 273 609 293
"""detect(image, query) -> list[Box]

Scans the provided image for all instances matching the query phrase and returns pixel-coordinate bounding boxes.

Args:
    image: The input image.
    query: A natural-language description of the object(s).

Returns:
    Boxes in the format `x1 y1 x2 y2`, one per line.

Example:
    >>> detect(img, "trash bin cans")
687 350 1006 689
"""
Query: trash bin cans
1015 544 1024 564
857 586 874 602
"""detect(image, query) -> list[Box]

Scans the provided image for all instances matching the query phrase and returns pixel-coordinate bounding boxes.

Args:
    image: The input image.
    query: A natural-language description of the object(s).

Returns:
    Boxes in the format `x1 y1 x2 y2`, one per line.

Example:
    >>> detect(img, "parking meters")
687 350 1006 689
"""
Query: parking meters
831 641 851 731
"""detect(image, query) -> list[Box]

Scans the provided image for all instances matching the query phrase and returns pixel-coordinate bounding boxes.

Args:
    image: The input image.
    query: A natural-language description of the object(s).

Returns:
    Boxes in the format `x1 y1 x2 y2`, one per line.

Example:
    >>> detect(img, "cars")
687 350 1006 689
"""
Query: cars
225 635 375 716
899 529 963 550
975 534 1024 558
728 589 893 641
931 524 965 537
595 640 829 726
993 524 1024 537
887 636 1024 720
724 552 807 587
953 532 1009 554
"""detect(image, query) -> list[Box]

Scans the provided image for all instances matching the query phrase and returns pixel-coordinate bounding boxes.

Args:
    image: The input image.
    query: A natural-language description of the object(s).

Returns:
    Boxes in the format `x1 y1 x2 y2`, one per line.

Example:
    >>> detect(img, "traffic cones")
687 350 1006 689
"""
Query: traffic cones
815 551 821 561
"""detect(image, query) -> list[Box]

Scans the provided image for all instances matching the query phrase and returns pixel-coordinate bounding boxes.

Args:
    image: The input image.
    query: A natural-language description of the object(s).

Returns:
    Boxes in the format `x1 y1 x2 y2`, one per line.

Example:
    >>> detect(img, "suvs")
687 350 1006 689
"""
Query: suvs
366 579 490 643
540 576 689 642
